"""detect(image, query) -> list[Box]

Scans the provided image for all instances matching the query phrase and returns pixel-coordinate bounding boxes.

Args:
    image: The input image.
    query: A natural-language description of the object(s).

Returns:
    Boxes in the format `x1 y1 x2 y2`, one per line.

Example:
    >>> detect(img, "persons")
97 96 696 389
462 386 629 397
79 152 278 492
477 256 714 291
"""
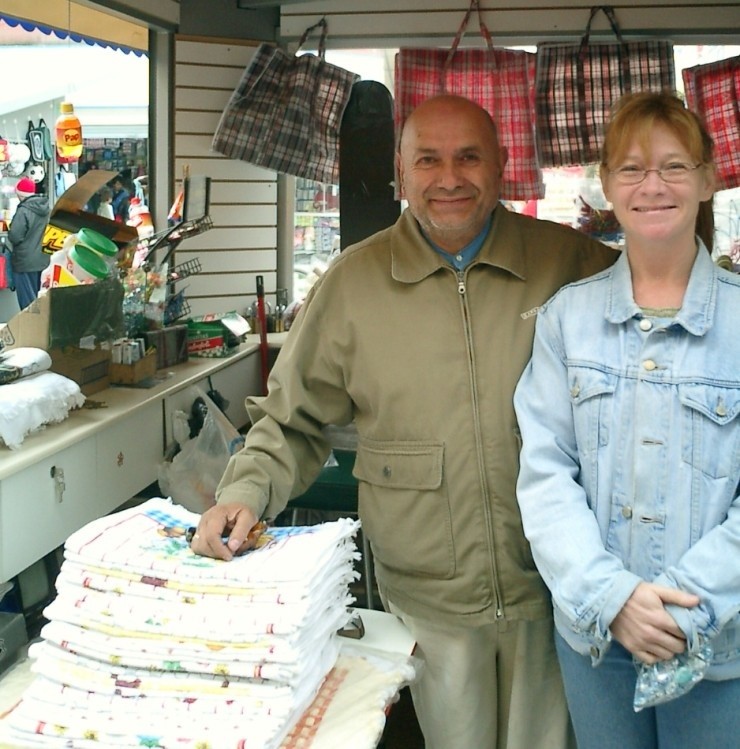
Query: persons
513 88 740 748
189 92 740 749
6 177 131 312
313 183 340 211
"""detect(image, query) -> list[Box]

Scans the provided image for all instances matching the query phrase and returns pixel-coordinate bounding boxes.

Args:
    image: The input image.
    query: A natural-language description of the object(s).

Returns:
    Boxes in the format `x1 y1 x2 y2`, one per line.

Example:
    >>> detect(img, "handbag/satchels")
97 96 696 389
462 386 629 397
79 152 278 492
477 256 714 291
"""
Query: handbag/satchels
25 118 53 164
155 384 248 516
536 7 675 167
391 0 549 201
211 19 359 186
680 57 740 193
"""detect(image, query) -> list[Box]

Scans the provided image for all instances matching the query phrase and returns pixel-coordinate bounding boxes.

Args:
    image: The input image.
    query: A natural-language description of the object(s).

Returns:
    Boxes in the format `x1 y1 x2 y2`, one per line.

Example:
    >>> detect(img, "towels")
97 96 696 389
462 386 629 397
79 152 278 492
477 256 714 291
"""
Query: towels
0 495 364 749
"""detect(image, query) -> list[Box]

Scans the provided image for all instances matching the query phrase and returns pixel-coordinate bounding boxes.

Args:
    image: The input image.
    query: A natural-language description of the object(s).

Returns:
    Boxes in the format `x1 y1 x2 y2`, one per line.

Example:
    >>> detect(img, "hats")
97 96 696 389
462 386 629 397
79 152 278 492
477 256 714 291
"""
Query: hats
14 177 36 197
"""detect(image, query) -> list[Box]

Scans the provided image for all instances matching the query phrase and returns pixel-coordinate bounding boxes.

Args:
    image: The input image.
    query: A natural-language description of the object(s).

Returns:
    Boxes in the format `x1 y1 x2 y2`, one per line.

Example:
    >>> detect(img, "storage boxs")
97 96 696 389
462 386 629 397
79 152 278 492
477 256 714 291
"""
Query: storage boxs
134 323 188 369
110 354 155 384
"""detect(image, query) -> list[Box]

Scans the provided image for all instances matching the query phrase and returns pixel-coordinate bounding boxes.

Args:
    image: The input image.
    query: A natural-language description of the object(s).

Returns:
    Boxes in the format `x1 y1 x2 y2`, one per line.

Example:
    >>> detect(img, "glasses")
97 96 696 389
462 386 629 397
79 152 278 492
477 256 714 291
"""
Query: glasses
608 161 692 185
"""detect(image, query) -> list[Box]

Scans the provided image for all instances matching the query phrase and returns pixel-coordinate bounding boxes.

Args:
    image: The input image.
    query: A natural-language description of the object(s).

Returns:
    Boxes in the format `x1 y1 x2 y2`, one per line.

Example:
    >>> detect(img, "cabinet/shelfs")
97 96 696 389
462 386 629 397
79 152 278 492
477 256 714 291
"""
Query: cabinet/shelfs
1 340 263 584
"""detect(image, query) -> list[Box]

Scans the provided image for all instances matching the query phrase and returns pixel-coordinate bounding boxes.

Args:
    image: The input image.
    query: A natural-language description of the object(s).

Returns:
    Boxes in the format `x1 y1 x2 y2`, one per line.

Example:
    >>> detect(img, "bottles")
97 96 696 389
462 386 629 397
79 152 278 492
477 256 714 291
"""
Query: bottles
63 227 118 272
55 103 83 158
61 244 108 286
130 198 154 237
